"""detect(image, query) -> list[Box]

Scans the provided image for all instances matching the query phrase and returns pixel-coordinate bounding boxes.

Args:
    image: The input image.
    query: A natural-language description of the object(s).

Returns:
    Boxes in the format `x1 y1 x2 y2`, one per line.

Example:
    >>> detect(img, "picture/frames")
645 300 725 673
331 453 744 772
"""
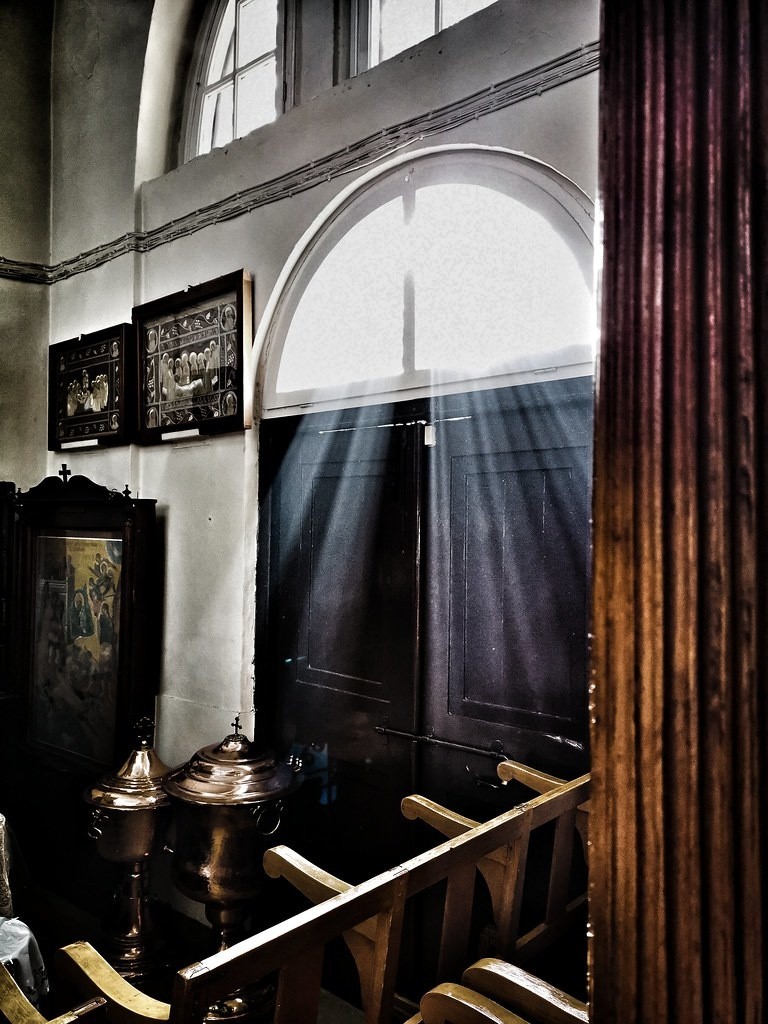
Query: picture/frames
130 267 254 448
48 323 135 451
0 463 156 779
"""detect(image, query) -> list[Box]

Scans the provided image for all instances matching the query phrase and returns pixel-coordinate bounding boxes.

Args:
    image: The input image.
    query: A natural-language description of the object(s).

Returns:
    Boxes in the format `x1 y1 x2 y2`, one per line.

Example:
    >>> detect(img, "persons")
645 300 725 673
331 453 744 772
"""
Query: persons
66 370 108 417
159 341 219 401
